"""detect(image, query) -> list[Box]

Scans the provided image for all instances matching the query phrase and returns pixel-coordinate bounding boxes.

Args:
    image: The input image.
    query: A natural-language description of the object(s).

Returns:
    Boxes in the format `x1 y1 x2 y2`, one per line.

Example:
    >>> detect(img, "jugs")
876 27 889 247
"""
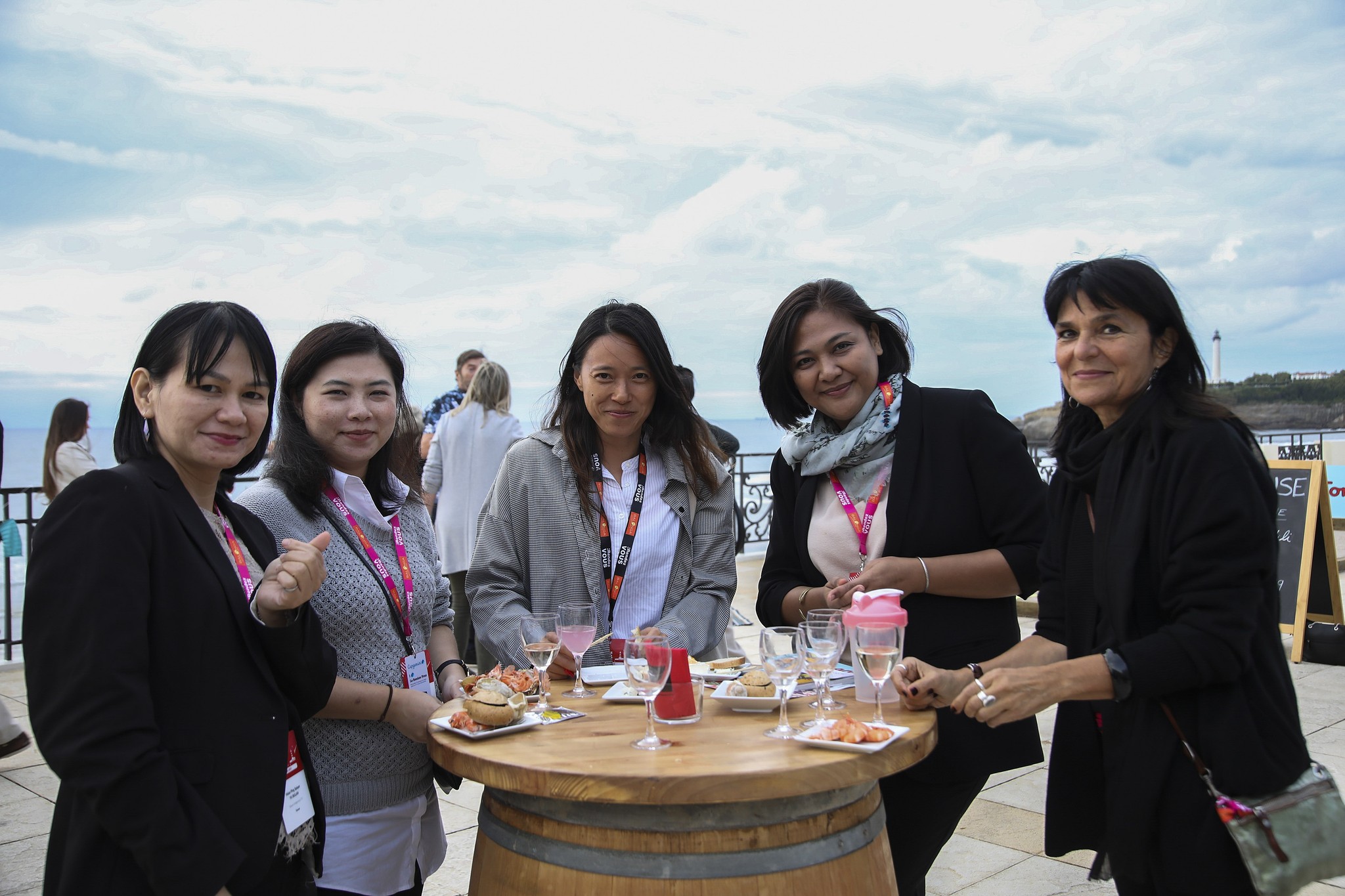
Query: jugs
842 589 907 703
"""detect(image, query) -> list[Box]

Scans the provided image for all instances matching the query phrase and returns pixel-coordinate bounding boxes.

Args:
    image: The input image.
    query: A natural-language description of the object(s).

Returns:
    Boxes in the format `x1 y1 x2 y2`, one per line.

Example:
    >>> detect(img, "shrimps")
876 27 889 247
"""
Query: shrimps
449 711 493 731
808 712 894 744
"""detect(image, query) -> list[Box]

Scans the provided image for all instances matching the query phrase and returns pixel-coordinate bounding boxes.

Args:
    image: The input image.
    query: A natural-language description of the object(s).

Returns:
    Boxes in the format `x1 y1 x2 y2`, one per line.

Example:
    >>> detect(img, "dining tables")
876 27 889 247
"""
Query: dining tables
424 662 939 895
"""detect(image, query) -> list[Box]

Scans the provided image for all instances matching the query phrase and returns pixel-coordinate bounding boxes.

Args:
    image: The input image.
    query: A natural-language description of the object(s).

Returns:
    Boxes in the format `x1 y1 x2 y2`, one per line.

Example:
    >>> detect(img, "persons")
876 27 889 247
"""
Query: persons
758 278 1067 896
22 304 342 896
418 348 486 506
888 251 1316 896
421 357 523 664
226 313 477 896
465 296 737 681
43 398 99 505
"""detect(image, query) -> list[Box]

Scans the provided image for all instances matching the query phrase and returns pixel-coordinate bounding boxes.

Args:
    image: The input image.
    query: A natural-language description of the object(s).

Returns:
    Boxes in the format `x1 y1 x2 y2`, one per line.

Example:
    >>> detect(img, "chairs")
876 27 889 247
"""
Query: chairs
687 550 794 664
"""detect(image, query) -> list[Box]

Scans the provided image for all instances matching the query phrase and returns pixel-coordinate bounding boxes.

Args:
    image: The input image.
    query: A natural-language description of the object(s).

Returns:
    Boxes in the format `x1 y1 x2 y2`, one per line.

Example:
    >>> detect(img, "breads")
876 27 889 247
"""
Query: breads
727 670 776 698
705 656 744 669
467 691 513 726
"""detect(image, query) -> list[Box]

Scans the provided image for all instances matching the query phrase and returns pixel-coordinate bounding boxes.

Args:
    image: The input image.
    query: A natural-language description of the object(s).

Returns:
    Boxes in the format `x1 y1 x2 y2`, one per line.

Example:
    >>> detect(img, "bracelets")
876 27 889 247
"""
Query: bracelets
964 663 983 680
376 684 393 724
434 659 470 695
799 587 814 621
916 556 929 594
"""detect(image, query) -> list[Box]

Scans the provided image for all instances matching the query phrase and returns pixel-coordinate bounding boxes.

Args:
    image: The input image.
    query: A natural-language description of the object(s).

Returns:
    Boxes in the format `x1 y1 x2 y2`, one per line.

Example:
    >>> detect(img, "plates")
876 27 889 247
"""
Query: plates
689 661 751 681
602 681 645 704
430 711 544 740
580 664 629 686
710 680 798 712
794 719 911 753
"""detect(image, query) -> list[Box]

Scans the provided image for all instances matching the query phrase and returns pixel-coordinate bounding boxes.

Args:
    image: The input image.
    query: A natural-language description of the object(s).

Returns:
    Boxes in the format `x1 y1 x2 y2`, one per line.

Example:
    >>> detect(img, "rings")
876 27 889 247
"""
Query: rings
284 586 298 593
976 691 996 706
893 664 908 673
975 678 985 691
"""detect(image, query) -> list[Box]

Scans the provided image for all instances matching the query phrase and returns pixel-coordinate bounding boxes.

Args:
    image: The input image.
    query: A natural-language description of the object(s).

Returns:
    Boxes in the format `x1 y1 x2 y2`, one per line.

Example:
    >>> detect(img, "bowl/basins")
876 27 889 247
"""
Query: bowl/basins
650 673 705 725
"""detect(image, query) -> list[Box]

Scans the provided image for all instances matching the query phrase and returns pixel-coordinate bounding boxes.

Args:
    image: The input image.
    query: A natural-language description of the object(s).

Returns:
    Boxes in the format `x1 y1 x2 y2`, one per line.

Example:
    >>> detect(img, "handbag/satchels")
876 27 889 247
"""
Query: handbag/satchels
1215 764 1345 896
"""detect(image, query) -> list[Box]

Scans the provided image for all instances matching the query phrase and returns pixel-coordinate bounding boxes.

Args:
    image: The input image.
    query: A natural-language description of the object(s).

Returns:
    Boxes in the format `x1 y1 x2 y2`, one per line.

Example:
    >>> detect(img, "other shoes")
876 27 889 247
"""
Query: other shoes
0 732 31 759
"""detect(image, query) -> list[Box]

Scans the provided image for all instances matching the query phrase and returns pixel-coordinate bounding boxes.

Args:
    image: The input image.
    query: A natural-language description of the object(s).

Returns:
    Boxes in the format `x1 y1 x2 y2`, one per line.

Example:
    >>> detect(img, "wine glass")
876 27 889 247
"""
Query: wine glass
520 603 597 713
624 636 672 752
759 608 900 740
